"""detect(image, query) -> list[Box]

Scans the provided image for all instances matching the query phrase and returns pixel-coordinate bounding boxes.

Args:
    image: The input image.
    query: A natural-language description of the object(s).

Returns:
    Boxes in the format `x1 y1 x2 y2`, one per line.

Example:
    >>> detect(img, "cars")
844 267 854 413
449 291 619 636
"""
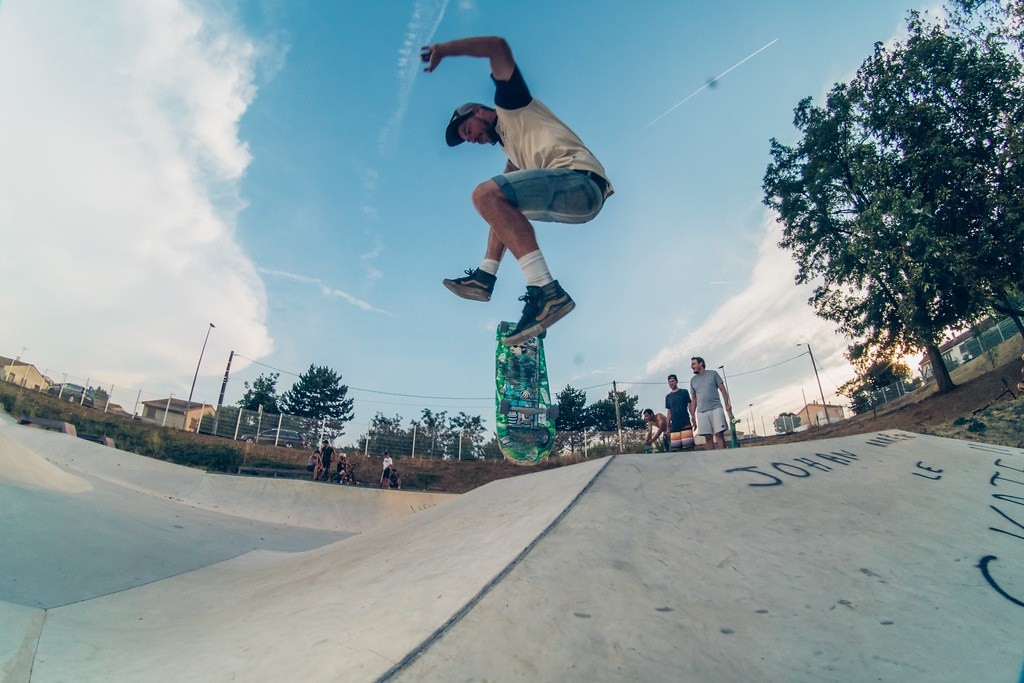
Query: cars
49 382 94 407
241 429 310 450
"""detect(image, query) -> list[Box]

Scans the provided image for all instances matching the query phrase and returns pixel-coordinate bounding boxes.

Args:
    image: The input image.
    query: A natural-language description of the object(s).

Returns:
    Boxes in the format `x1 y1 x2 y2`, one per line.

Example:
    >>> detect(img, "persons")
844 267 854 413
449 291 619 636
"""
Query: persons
307 440 402 490
644 357 732 451
422 36 614 347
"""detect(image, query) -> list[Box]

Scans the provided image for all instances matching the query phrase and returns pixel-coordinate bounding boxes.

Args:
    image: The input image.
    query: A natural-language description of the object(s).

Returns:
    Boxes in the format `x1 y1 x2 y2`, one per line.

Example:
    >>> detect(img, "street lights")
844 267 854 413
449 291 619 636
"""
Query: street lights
796 343 830 424
182 322 216 427
719 365 739 448
749 403 756 436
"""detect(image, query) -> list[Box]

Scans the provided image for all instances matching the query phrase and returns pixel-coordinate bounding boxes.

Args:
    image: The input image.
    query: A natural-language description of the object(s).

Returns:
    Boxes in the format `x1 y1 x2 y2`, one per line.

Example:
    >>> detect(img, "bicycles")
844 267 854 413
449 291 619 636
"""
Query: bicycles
331 462 360 486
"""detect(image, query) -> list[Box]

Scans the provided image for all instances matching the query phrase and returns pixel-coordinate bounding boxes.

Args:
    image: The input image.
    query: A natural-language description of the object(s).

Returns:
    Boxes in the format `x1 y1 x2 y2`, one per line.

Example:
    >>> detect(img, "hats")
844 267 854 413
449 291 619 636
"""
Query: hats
445 102 487 147
668 375 678 383
384 452 388 455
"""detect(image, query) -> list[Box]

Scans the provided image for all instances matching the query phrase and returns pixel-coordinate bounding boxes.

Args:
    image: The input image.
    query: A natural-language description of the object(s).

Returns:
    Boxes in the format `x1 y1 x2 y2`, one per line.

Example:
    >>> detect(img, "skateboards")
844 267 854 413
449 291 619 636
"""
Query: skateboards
495 321 551 465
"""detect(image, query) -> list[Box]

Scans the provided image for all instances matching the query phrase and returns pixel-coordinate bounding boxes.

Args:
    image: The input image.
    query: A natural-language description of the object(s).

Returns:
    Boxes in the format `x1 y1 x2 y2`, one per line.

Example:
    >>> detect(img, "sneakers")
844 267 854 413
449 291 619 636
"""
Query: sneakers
442 267 497 302
504 279 576 346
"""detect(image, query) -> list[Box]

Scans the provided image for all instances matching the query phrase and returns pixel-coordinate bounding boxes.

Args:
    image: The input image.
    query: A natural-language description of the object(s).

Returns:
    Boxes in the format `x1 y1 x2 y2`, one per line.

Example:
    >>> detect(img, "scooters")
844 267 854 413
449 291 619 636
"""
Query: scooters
972 378 1016 416
